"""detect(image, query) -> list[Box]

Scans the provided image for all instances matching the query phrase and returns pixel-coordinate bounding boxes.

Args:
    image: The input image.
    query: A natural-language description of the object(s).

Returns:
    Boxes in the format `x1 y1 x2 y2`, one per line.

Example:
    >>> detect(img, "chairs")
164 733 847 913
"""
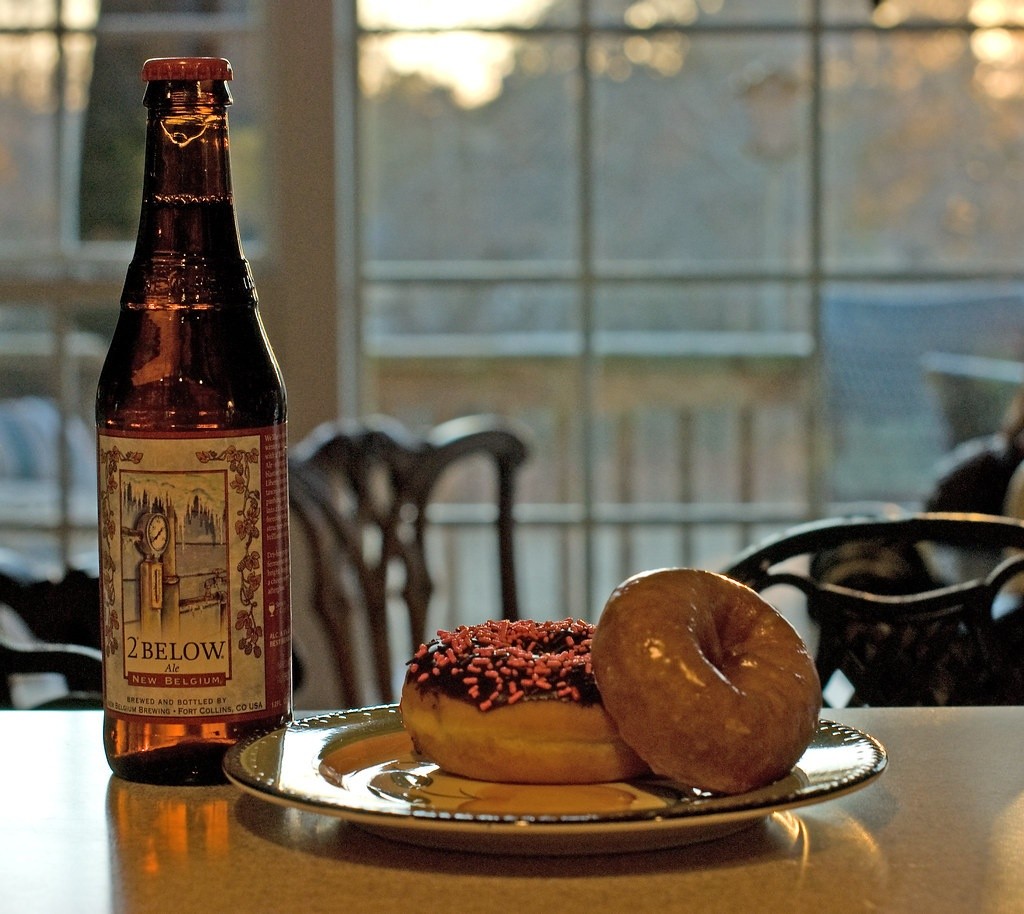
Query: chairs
290 413 1024 706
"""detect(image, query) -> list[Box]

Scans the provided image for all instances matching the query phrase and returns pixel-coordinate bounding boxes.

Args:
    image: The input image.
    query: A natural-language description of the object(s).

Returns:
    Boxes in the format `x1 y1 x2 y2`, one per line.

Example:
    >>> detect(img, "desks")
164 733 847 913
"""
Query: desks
0 710 1024 914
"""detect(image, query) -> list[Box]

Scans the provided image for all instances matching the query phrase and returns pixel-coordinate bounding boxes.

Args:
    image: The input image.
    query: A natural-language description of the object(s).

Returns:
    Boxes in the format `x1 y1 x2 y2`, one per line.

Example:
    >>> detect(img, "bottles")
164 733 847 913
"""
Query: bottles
97 55 289 782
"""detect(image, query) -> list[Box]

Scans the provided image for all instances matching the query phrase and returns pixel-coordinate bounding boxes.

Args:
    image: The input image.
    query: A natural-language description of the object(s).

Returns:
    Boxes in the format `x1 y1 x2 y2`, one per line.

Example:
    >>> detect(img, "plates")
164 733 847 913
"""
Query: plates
226 701 884 851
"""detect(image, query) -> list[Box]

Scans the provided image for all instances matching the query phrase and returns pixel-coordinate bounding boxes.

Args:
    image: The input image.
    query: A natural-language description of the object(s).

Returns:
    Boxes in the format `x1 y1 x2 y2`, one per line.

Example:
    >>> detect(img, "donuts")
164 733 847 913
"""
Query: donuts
589 568 822 791
399 618 642 785
